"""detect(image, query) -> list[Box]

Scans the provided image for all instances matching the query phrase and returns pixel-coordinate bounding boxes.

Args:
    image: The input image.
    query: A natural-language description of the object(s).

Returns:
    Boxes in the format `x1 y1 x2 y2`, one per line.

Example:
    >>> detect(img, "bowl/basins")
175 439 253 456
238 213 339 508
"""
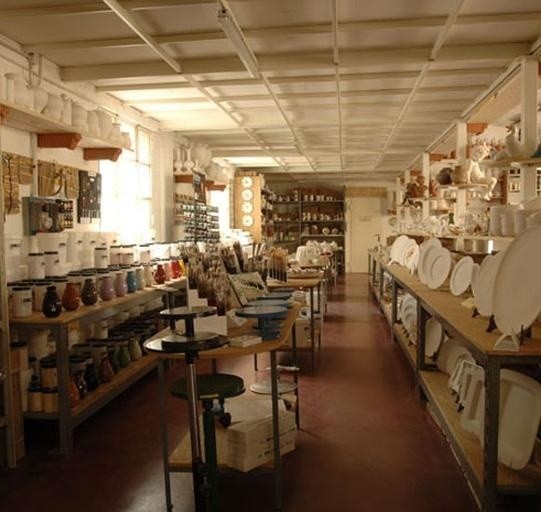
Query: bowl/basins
489 205 531 236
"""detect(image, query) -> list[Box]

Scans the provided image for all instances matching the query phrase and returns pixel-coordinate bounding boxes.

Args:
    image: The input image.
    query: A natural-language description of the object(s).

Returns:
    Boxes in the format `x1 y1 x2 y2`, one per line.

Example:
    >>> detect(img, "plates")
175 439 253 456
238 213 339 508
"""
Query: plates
449 257 474 295
497 368 541 469
474 255 494 317
398 292 418 348
388 234 420 275
437 339 475 395
425 318 442 357
492 226 541 333
460 370 486 441
417 236 441 283
424 248 450 290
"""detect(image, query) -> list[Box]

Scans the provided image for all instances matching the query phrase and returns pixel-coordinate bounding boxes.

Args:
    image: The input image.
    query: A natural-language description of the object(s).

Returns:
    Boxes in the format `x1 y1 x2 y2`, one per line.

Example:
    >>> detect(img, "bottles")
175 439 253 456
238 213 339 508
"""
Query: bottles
138 268 146 291
98 275 116 301
116 272 128 298
43 286 61 317
128 269 139 294
155 264 165 284
63 282 80 312
82 279 98 308
145 264 155 286
13 287 34 319
32 282 52 311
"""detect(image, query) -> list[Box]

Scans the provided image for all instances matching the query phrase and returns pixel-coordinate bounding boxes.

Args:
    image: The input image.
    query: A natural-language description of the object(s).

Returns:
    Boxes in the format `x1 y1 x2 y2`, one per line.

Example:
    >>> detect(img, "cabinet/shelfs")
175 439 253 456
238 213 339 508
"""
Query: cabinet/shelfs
367 251 541 512
174 174 226 245
263 188 345 276
9 278 187 451
142 300 302 512
384 55 541 269
252 277 324 373
